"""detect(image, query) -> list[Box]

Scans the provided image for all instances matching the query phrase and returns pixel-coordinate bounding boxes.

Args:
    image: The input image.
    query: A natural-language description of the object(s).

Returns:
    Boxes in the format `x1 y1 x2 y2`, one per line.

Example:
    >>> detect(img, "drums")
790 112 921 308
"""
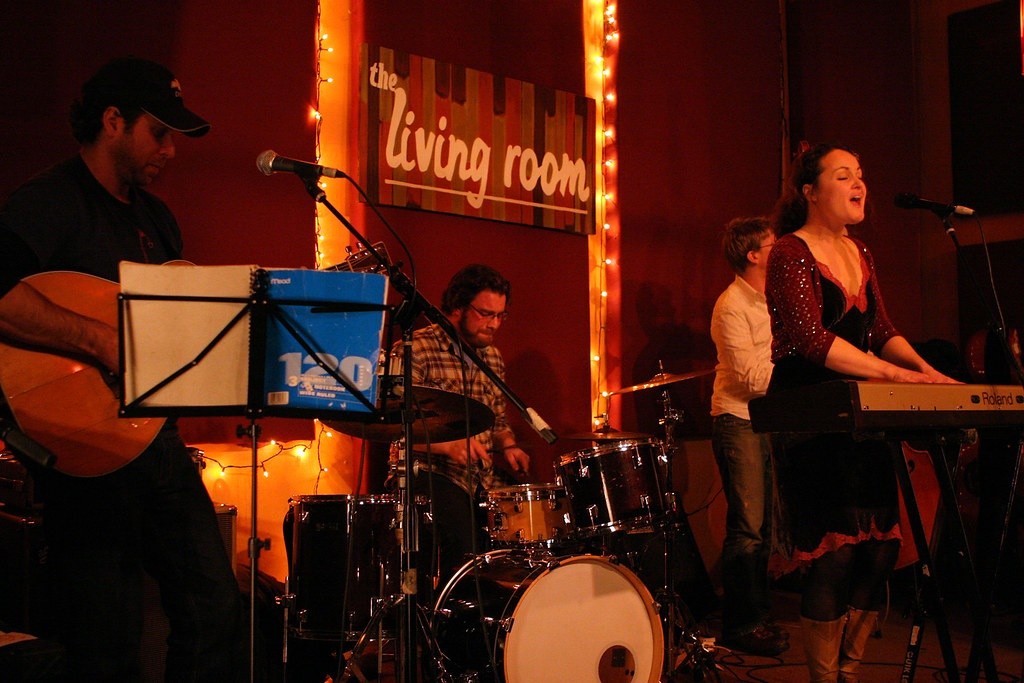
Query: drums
283 494 440 643
480 483 580 547
428 547 664 683
555 437 677 541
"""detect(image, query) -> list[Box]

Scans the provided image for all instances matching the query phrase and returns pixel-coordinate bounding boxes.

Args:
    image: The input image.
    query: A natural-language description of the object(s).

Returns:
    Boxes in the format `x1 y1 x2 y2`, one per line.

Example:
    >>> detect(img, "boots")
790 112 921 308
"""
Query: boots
839 603 880 683
796 609 848 683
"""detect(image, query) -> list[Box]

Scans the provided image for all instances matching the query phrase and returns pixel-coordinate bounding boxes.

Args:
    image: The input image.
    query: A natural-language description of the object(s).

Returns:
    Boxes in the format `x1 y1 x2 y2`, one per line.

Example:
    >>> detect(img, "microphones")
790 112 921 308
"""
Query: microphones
256 150 346 178
894 191 975 215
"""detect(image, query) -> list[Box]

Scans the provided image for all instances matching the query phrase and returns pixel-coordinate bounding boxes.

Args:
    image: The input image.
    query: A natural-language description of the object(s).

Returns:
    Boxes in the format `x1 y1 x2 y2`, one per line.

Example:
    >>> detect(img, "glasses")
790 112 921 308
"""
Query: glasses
468 301 510 322
752 239 777 252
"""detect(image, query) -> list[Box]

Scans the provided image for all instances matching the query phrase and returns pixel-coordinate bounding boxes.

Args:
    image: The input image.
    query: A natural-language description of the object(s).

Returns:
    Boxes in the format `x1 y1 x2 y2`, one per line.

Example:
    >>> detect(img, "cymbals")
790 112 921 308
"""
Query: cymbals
606 368 720 394
317 381 497 444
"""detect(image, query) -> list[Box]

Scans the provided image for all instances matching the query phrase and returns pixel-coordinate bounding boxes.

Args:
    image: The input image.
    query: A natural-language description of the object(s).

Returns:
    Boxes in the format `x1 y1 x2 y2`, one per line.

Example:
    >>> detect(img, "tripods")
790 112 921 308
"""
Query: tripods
339 409 450 683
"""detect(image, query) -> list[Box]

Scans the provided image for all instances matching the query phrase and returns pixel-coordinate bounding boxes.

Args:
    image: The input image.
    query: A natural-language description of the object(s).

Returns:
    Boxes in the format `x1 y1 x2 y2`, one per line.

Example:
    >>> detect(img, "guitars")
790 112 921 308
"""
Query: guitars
0 238 397 479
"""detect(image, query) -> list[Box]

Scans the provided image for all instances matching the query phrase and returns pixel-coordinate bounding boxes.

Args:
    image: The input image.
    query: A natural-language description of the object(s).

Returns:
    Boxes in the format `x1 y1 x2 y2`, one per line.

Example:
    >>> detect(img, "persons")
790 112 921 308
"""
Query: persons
0 54 241 683
764 142 974 683
387 263 531 586
708 217 790 657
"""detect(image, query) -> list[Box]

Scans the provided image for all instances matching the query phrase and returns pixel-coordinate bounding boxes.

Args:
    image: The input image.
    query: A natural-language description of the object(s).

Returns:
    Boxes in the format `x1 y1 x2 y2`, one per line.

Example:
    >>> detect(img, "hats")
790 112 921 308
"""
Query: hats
82 53 212 138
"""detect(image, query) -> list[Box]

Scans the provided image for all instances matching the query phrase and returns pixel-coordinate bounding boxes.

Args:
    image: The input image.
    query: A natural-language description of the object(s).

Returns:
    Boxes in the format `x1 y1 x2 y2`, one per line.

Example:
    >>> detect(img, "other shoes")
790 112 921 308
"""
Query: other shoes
763 621 790 641
716 622 790 658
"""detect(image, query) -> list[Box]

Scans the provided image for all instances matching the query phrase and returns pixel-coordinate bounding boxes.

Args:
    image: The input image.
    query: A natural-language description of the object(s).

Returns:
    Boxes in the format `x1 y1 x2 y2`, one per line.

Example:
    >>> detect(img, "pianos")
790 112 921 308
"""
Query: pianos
748 378 1024 683
